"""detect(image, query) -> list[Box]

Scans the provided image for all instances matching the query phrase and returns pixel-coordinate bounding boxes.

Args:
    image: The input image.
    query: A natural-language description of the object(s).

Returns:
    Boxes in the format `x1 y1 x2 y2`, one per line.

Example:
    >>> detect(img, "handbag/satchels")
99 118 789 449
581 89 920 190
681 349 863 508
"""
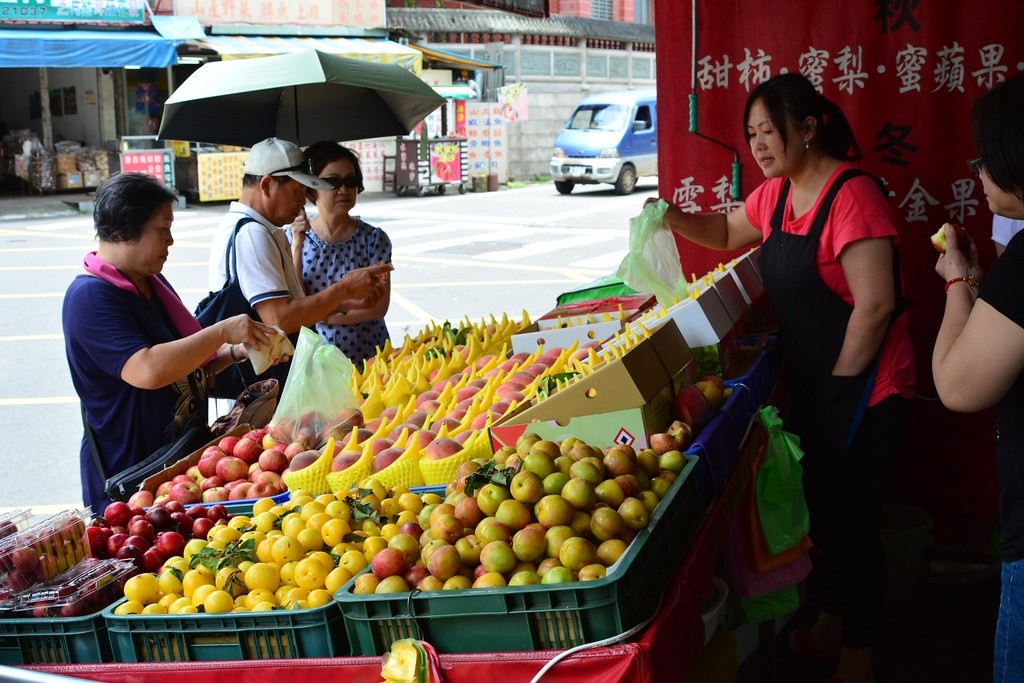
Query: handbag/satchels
103 414 214 502
194 217 318 401
211 378 279 438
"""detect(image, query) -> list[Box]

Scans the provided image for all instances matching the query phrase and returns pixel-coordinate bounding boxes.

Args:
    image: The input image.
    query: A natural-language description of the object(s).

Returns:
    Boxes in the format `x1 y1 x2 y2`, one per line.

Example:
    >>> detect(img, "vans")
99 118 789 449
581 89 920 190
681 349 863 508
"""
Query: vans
550 90 659 195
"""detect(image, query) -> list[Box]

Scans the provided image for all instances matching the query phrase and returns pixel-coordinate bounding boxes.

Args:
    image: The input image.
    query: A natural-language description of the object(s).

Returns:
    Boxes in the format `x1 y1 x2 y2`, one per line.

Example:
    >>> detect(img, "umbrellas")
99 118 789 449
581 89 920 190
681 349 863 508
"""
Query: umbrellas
156 49 450 209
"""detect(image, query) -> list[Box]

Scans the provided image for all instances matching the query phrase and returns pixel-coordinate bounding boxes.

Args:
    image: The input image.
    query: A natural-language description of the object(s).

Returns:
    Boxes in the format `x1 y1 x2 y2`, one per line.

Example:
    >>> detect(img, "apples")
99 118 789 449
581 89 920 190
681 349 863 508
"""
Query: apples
125 409 361 504
692 375 724 410
931 223 969 254
354 421 694 594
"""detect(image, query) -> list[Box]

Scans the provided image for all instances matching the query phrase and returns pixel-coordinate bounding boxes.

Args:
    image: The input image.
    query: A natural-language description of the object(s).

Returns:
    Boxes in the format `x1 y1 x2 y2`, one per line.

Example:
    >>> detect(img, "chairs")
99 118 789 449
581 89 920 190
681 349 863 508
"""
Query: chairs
383 155 396 193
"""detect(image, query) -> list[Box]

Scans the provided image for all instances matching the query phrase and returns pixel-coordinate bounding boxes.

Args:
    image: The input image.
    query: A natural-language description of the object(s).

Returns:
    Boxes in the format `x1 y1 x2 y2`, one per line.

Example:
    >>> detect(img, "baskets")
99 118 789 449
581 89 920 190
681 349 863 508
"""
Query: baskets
0 345 774 667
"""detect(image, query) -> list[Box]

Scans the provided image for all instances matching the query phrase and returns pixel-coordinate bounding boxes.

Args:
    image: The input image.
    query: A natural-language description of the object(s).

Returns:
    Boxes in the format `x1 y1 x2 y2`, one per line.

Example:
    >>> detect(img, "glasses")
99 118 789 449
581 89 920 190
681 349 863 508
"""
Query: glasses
966 157 983 176
269 159 314 175
321 175 359 189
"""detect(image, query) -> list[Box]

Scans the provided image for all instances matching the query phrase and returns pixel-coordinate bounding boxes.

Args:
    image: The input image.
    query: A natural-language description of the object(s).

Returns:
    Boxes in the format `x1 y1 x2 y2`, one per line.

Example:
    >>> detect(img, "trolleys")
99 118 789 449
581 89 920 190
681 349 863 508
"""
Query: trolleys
394 128 469 197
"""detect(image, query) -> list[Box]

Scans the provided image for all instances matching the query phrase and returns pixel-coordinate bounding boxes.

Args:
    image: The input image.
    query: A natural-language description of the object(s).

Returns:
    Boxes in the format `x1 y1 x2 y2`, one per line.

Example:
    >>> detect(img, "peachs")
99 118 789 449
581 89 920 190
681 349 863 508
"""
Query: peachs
672 383 709 427
291 325 599 476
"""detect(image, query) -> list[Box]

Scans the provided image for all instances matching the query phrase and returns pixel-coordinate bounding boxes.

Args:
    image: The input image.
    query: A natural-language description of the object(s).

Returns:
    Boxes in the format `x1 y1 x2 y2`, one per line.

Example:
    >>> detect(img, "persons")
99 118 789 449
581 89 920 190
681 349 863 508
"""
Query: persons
209 138 393 406
932 76 1024 683
281 143 396 375
64 171 291 522
643 72 917 683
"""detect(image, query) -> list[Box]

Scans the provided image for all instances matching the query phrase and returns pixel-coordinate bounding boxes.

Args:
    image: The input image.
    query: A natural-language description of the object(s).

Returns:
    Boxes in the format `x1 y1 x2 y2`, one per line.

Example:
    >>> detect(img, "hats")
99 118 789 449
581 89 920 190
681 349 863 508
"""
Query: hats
242 137 335 190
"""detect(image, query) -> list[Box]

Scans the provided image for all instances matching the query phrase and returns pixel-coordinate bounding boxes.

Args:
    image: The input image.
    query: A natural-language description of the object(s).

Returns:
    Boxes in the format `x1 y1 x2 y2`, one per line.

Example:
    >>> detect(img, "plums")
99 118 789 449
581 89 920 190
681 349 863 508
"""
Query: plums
0 479 442 618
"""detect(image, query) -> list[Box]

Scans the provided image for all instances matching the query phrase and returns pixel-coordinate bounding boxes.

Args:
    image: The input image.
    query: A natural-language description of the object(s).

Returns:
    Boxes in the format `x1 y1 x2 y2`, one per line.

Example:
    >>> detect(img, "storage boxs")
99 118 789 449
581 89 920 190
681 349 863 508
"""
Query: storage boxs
1 247 764 662
57 172 82 188
82 170 101 187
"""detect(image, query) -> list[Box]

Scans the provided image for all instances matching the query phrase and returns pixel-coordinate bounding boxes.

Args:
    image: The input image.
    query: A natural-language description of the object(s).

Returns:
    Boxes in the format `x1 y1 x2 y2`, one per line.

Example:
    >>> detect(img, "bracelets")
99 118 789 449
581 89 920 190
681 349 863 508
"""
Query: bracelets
945 276 979 292
230 344 247 363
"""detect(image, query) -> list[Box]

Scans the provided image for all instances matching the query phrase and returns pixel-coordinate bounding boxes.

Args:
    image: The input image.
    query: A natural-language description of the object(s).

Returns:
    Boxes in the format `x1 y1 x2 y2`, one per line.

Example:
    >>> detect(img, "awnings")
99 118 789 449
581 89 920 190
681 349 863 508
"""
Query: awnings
192 40 423 78
0 30 179 68
408 41 506 71
